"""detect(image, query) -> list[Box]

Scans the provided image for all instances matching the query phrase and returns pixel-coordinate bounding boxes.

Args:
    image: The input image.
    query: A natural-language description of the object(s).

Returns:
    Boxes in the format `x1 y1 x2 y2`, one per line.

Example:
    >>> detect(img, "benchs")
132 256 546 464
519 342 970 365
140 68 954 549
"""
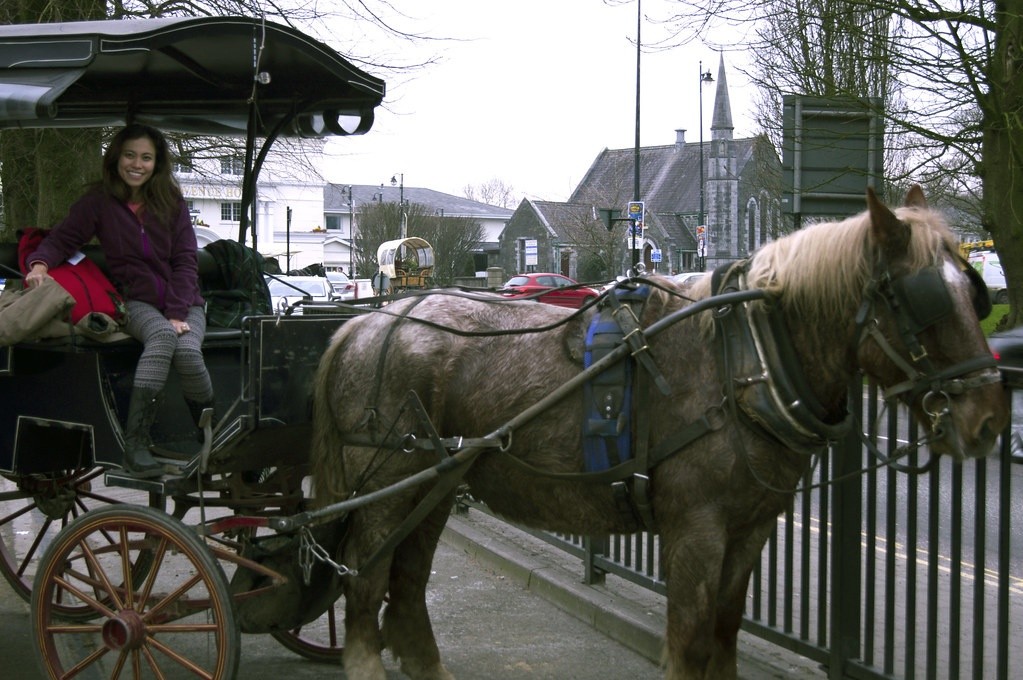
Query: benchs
395 269 407 286
0 243 253 353
408 268 429 286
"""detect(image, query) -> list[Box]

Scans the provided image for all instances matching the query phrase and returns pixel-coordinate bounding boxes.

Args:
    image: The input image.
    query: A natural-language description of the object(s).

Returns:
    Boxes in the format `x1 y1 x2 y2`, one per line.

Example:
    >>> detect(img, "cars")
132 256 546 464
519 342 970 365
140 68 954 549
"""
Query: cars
261 271 389 316
499 273 707 316
985 327 1023 462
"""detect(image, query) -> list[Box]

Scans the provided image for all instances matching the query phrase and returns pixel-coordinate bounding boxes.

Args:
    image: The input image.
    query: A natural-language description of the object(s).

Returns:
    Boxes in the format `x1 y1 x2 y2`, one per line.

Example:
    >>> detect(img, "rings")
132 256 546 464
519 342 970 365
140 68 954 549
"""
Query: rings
182 325 186 327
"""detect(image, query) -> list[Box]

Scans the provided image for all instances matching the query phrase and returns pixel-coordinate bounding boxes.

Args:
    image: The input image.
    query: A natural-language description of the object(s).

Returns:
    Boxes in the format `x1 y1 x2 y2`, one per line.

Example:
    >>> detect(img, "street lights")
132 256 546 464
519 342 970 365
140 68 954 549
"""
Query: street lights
371 182 384 243
700 58 716 269
342 185 353 279
390 171 409 262
433 208 444 238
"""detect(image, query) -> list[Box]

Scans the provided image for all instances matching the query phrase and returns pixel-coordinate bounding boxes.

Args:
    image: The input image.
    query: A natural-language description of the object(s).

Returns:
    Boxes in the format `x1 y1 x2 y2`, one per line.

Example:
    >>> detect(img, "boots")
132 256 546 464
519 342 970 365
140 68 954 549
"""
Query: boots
121 384 167 479
184 393 221 446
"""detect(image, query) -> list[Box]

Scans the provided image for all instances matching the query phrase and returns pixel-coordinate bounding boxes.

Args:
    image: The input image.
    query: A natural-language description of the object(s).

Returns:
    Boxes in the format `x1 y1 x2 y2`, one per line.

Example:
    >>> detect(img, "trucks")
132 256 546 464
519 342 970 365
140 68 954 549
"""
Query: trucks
958 240 1006 305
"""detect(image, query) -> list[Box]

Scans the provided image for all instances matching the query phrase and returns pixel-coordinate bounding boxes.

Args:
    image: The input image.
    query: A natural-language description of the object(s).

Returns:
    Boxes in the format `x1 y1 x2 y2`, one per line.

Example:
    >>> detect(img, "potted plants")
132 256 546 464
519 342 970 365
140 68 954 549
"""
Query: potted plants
310 225 327 232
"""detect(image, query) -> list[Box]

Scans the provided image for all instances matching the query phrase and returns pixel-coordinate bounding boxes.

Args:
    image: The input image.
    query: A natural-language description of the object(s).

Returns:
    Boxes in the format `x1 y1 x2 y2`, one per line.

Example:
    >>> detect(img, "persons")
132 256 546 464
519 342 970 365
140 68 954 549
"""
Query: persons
24 123 215 479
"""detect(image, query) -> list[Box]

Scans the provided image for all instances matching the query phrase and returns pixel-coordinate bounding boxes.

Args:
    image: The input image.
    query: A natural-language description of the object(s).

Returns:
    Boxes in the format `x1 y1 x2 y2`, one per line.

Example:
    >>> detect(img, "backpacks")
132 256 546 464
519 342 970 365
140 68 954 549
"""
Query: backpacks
13 223 129 323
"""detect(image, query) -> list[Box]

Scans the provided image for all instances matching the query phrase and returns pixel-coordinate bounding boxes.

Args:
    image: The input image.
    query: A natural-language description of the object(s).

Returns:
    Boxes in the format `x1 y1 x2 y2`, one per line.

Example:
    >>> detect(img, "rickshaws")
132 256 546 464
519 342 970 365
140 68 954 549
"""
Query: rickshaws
377 236 435 296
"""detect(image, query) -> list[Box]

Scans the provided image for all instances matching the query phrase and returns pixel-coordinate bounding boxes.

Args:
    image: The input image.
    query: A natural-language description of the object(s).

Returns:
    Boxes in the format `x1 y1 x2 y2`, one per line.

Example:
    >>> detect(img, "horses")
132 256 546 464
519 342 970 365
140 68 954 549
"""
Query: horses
299 184 1013 680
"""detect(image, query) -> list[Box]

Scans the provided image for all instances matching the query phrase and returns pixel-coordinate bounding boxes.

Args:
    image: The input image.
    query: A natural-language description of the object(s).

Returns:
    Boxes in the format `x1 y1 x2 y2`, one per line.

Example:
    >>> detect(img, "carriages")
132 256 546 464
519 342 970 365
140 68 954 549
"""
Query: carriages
0 17 1006 680
